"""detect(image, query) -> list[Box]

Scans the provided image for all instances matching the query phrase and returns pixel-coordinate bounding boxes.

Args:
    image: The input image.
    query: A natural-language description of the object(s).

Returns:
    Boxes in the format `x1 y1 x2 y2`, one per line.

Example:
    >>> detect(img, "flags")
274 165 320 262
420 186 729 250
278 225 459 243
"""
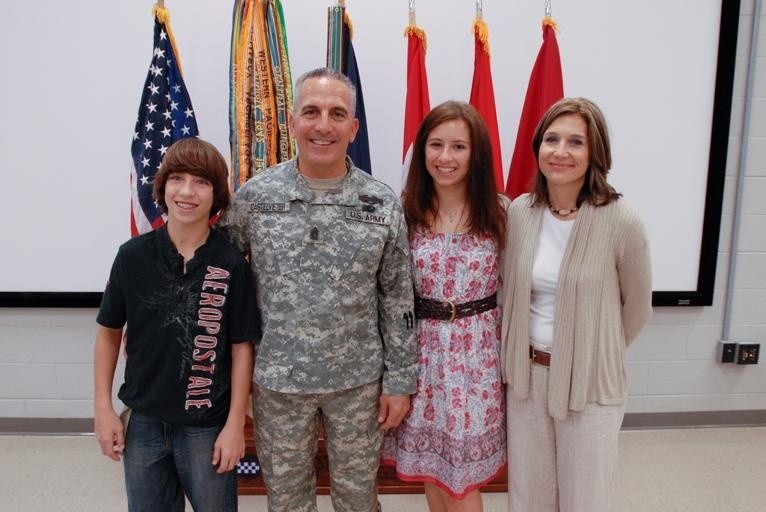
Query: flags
469 23 506 195
228 2 300 421
505 20 564 203
325 6 371 175
400 25 432 190
130 6 201 238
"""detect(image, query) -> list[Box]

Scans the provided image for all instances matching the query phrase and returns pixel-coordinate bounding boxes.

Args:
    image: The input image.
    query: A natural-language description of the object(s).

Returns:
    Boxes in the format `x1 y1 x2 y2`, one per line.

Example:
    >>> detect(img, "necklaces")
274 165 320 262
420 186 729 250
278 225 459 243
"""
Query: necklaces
543 189 581 218
446 211 457 225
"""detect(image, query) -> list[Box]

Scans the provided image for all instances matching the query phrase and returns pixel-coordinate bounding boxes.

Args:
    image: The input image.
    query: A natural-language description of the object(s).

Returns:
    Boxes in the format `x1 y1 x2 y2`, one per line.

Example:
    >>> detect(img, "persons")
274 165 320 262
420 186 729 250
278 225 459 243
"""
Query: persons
216 66 420 511
90 139 264 511
380 99 513 511
498 97 655 512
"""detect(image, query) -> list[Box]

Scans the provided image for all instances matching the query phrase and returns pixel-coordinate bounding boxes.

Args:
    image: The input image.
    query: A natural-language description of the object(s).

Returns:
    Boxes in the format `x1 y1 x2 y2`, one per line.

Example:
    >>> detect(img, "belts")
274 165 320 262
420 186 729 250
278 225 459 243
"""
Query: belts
528 346 550 366
414 292 496 323
